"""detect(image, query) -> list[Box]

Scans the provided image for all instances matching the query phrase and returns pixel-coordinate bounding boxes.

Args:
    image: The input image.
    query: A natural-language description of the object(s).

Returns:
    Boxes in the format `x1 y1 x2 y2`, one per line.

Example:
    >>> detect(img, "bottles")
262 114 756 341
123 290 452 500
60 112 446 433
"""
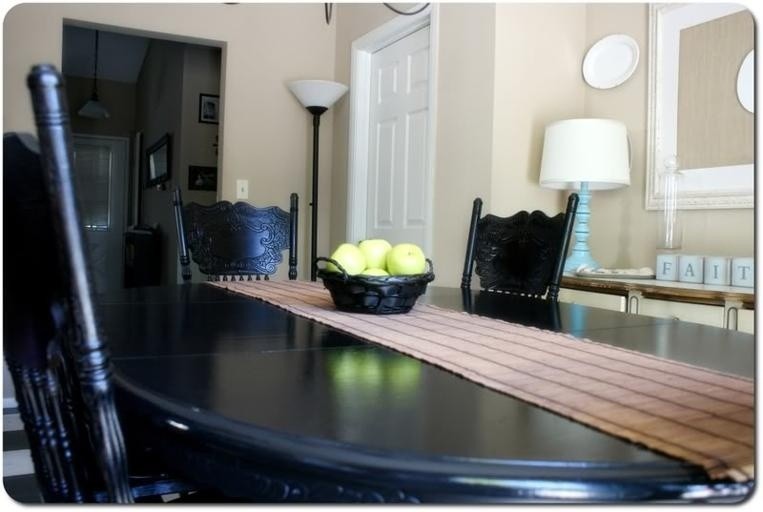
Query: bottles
654 153 685 252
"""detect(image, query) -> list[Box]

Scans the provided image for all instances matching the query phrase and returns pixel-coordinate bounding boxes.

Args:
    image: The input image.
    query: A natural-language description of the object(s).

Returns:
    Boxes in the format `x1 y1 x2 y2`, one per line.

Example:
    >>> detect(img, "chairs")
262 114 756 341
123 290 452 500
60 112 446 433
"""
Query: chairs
459 188 580 303
169 186 303 282
8 63 208 502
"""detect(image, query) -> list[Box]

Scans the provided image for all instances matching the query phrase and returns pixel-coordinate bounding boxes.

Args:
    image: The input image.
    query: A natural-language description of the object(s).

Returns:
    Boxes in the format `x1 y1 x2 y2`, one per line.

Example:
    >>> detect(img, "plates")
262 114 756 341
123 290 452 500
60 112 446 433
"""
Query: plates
582 33 640 91
734 47 754 115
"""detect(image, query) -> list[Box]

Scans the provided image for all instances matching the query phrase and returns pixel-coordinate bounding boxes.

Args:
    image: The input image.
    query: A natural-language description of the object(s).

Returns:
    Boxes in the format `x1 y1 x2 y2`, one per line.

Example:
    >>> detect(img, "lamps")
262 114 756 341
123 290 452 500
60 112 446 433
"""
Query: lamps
282 75 353 276
539 118 631 276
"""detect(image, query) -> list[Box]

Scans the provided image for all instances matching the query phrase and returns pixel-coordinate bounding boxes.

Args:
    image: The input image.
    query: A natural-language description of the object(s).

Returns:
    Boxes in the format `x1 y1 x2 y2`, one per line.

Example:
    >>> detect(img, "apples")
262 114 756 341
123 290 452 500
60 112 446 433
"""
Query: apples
326 244 368 275
362 269 389 275
386 243 426 275
358 239 392 270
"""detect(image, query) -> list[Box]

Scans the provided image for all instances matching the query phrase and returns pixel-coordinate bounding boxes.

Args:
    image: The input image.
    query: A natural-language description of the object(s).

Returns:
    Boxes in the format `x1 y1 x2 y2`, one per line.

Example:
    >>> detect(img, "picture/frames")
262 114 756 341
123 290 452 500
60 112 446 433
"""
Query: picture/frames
143 93 220 192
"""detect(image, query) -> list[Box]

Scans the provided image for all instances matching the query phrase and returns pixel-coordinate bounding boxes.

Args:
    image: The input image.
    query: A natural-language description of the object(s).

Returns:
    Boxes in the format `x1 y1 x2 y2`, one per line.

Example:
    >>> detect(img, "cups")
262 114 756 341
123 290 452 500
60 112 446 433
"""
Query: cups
729 256 756 289
654 254 678 282
676 255 704 284
702 255 732 286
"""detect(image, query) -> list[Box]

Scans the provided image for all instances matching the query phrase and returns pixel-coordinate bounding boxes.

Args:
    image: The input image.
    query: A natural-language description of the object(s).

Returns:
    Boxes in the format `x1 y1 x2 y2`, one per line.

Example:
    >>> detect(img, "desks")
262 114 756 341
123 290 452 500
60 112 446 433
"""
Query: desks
540 273 755 335
95 280 755 505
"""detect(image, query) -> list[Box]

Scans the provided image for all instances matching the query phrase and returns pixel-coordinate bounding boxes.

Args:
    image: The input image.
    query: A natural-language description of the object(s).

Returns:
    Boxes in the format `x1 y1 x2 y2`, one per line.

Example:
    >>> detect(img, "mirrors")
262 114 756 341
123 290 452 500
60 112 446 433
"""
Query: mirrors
643 2 755 211
